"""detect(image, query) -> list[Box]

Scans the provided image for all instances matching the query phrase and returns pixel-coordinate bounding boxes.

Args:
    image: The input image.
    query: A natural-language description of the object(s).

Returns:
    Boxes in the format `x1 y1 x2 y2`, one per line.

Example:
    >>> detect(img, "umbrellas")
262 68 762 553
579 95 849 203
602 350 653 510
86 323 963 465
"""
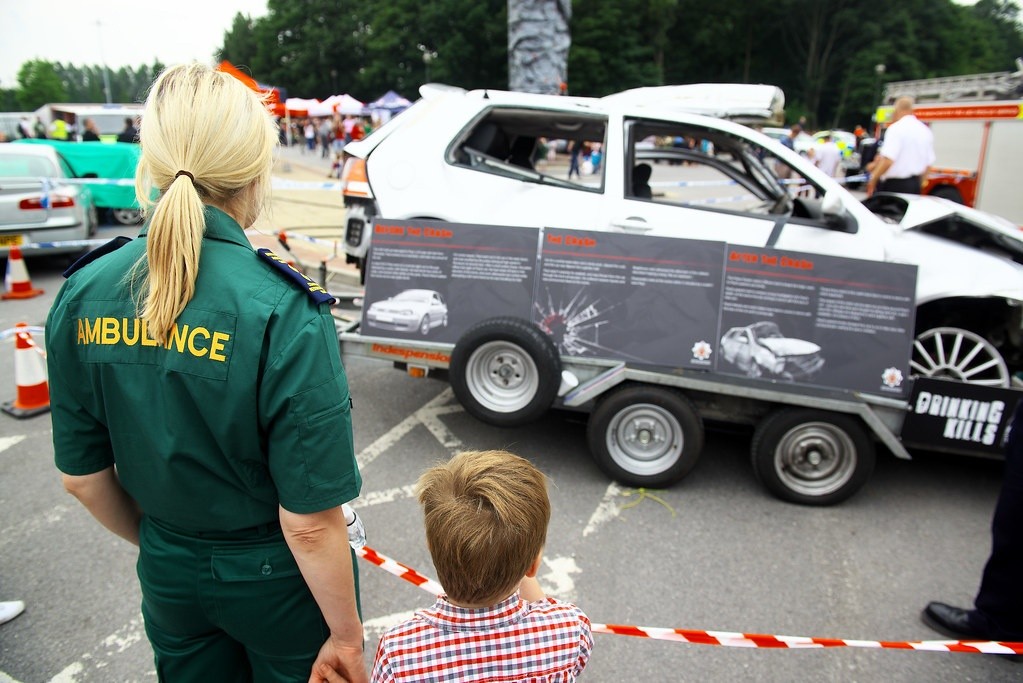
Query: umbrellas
215 59 412 148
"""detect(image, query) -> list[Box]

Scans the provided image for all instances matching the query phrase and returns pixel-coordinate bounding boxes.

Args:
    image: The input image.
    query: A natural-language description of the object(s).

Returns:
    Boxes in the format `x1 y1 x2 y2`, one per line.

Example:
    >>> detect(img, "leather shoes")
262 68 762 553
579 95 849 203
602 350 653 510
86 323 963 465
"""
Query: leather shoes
921 603 1023 664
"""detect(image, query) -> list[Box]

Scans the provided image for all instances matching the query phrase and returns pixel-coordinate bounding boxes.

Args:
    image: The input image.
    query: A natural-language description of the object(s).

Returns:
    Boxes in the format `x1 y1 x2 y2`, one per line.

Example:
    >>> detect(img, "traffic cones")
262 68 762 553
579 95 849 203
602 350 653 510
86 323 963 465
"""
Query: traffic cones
276 232 296 267
0 322 53 420
0 243 45 300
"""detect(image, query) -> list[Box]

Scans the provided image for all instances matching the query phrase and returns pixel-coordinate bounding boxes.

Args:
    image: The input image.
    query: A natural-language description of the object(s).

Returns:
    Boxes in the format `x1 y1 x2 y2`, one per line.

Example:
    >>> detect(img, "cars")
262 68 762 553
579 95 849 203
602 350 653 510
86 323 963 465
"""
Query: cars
330 83 1022 505
10 137 154 227
0 142 99 268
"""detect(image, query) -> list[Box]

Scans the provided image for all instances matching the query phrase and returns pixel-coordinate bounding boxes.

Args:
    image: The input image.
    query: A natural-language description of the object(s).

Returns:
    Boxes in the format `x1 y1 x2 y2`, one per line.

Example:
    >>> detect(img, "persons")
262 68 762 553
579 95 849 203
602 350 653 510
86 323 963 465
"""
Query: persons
920 403 1023 664
117 115 141 143
655 135 715 166
861 95 936 198
81 118 100 141
45 65 369 683
321 449 595 683
747 124 870 199
275 113 382 179
16 114 78 141
532 137 603 181
